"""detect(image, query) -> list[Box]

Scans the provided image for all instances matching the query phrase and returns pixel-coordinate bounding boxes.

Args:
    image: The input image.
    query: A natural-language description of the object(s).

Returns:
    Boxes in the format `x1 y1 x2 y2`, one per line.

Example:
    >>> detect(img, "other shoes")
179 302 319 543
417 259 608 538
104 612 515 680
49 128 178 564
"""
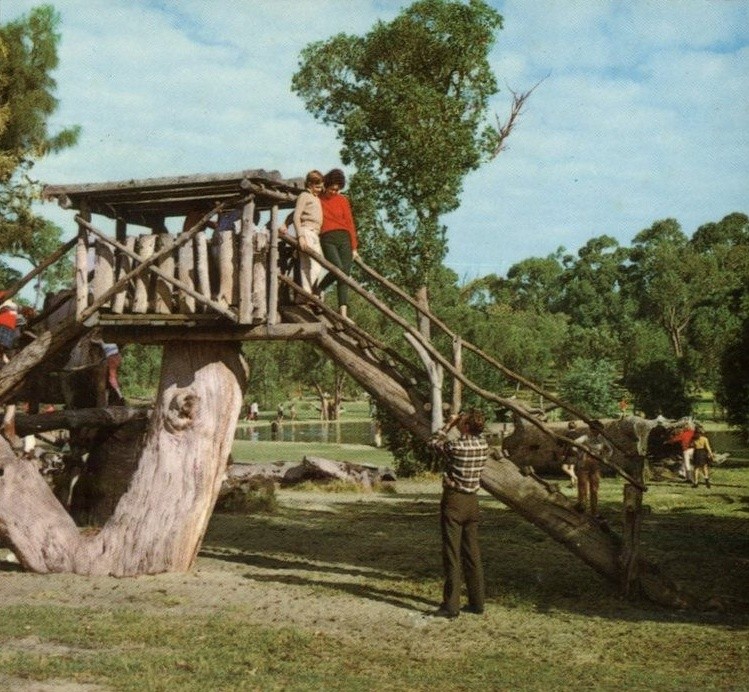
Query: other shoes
574 476 578 487
427 607 459 617
461 605 484 614
705 477 711 489
690 482 699 488
566 482 575 489
592 510 600 517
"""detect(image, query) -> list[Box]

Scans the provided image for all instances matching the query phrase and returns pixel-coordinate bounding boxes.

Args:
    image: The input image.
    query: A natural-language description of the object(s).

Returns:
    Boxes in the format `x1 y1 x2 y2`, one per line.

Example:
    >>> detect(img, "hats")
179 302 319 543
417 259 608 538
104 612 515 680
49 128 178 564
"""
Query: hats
694 423 706 433
589 419 605 430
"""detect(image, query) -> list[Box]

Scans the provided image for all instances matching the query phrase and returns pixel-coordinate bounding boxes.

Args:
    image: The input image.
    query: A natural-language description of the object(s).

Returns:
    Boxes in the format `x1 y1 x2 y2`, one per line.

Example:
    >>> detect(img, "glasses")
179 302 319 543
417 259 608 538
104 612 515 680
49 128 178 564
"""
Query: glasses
329 189 339 192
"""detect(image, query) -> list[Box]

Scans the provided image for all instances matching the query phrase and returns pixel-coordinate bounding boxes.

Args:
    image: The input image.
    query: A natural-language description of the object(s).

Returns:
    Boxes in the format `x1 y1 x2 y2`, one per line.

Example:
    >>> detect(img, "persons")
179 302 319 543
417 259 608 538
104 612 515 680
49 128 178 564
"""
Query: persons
428 407 489 618
244 400 258 422
290 403 295 421
183 211 242 293
573 419 613 521
664 422 714 489
276 403 283 423
0 269 124 452
557 421 579 487
370 407 381 446
280 169 361 317
293 170 325 304
